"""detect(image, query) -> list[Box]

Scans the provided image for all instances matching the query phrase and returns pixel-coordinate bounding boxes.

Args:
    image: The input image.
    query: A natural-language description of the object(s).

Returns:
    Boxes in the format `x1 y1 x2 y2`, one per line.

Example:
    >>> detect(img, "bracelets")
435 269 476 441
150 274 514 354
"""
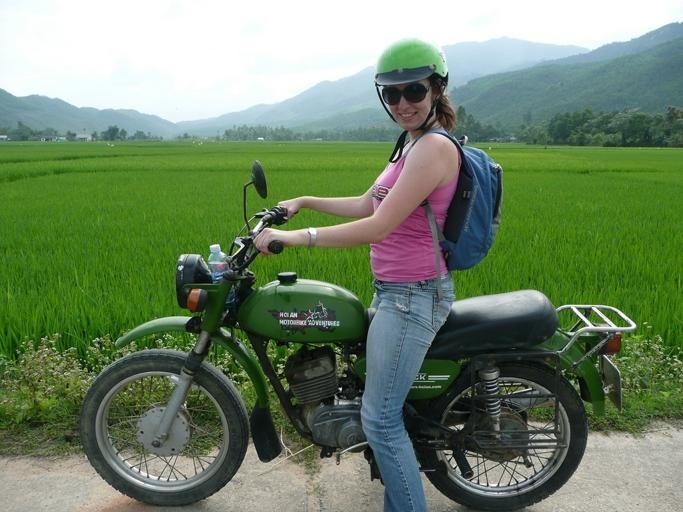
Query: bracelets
306 225 318 249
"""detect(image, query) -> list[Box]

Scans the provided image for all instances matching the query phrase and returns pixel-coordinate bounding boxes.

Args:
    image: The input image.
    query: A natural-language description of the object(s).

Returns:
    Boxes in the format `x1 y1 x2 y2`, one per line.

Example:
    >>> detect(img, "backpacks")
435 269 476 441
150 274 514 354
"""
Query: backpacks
418 127 504 271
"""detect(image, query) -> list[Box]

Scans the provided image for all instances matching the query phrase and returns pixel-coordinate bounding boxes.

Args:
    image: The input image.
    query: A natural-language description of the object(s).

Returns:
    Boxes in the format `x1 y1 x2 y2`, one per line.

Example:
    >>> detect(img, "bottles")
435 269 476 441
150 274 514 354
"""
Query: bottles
206 244 235 304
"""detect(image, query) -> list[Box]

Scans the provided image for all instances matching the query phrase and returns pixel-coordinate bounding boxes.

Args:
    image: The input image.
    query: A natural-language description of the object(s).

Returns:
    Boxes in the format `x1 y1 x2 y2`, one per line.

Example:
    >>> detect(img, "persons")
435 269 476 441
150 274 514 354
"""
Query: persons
247 37 463 511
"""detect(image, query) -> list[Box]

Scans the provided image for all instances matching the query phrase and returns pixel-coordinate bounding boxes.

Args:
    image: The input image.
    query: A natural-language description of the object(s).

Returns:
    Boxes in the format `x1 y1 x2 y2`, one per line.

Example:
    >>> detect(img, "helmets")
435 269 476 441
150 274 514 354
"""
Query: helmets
374 38 448 86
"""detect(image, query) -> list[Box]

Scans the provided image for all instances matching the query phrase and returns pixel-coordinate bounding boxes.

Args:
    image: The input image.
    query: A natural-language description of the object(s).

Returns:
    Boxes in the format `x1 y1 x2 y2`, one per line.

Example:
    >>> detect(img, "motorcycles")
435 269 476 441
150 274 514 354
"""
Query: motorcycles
80 158 638 511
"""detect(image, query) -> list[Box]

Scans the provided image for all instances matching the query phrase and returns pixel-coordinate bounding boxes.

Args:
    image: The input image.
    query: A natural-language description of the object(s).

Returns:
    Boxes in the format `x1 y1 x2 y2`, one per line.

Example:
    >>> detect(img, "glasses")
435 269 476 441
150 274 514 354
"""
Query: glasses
382 82 430 104
